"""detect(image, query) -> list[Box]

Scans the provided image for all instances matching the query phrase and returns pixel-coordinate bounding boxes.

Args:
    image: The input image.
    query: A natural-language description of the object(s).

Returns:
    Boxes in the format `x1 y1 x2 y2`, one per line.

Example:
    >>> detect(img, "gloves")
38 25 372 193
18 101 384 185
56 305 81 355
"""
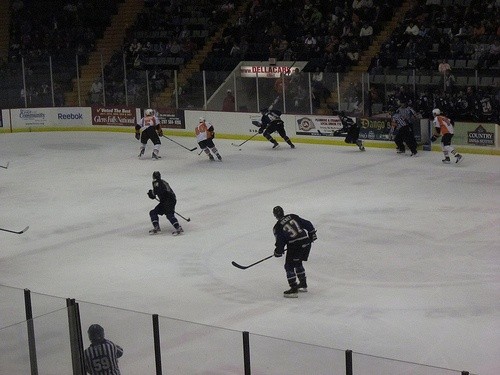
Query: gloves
430 135 438 142
258 128 264 134
135 132 140 140
308 231 317 242
158 130 164 137
147 190 156 199
274 248 285 257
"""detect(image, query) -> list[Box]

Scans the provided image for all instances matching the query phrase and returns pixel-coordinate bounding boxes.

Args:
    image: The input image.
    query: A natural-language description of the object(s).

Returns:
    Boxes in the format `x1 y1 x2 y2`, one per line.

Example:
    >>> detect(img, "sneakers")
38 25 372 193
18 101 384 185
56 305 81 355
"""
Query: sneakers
272 142 279 149
172 225 184 235
210 155 216 161
298 283 308 292
355 139 464 166
149 227 162 234
217 155 223 160
283 287 299 298
138 147 144 157
291 144 296 149
152 152 162 159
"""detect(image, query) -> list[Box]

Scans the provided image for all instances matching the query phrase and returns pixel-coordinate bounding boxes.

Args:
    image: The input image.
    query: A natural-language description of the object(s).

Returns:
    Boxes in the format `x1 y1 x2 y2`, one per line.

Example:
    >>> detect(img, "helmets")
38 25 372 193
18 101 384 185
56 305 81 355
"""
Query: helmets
199 117 206 123
87 324 105 343
273 206 284 218
260 107 268 115
432 108 441 116
152 171 161 181
145 109 154 116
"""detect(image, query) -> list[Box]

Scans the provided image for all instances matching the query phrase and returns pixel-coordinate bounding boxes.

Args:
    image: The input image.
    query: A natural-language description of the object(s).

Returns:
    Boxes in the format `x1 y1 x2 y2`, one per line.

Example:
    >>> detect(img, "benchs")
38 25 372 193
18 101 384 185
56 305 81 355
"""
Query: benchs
0 0 500 123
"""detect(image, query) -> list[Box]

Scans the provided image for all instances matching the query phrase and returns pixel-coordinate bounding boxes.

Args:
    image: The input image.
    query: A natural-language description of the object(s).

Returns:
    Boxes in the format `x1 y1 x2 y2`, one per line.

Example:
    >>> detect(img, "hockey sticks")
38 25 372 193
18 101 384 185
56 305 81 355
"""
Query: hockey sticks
155 198 191 222
232 249 287 270
198 150 203 156
317 129 347 136
232 132 259 147
0 162 9 169
418 140 433 146
0 225 30 234
162 134 197 151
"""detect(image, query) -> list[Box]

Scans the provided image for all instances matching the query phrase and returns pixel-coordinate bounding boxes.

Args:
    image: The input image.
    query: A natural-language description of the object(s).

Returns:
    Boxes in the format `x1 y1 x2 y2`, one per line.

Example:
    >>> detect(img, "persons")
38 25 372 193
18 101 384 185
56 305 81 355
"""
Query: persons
135 109 163 159
389 108 418 157
272 206 317 298
335 111 364 151
83 323 123 375
0 0 500 124
194 117 222 160
149 171 184 235
431 108 464 165
397 97 422 151
258 105 296 150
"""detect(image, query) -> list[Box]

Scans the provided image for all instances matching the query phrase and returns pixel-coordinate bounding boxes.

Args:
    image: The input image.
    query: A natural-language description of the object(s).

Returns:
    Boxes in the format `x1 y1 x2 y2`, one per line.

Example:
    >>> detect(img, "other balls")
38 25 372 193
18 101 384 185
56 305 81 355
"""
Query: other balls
238 149 242 151
